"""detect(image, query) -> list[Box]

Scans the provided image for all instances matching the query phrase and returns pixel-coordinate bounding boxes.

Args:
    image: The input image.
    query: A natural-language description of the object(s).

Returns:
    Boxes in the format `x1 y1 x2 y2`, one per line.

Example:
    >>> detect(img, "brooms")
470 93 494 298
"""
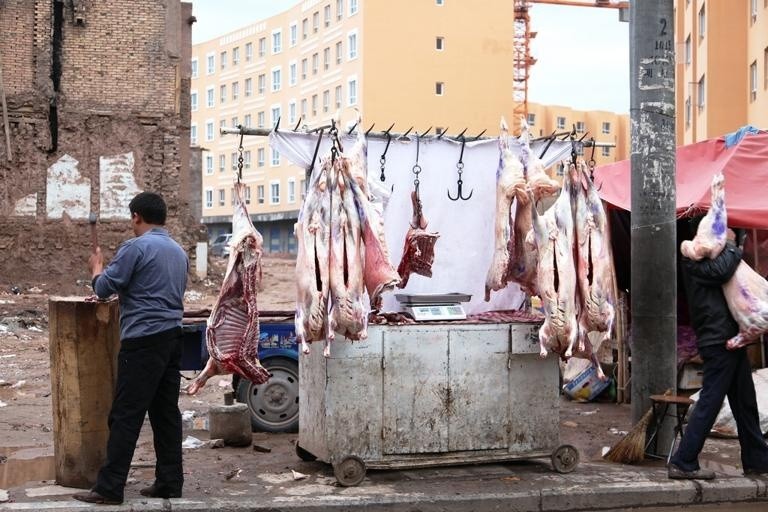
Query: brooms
607 391 672 465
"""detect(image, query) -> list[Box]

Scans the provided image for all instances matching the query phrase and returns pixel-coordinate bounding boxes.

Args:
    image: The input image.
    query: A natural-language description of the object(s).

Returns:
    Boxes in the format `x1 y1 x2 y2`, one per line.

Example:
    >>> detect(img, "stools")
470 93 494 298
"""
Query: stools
644 393 695 466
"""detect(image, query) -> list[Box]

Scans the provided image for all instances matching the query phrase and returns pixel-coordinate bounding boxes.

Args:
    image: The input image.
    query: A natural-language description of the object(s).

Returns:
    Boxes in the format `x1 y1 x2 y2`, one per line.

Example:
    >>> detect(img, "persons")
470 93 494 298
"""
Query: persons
69 189 192 506
665 210 767 481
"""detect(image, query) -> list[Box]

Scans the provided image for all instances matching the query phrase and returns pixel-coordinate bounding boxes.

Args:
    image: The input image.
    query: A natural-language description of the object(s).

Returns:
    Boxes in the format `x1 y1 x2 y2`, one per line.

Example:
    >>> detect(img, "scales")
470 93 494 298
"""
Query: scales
396 292 471 322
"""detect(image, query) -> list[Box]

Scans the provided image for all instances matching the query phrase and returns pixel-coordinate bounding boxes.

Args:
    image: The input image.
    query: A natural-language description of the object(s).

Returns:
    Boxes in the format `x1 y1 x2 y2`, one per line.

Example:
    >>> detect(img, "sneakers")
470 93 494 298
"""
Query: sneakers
667 460 717 480
72 489 124 505
139 481 182 499
